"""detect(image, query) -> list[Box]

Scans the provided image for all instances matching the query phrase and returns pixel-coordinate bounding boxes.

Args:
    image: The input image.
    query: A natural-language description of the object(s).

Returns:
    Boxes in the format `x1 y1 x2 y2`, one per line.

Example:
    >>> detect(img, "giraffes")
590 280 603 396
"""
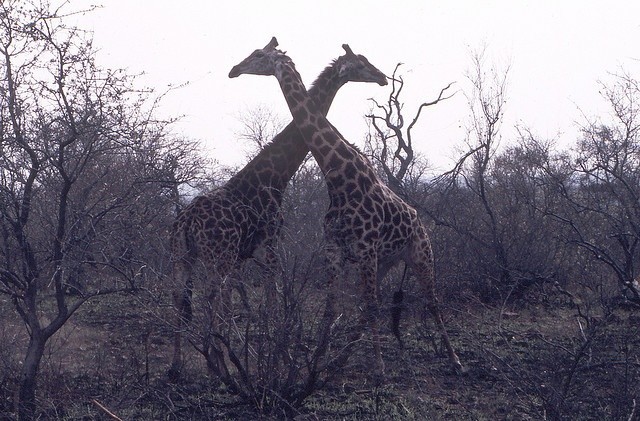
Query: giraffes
226 35 469 387
164 43 389 396
